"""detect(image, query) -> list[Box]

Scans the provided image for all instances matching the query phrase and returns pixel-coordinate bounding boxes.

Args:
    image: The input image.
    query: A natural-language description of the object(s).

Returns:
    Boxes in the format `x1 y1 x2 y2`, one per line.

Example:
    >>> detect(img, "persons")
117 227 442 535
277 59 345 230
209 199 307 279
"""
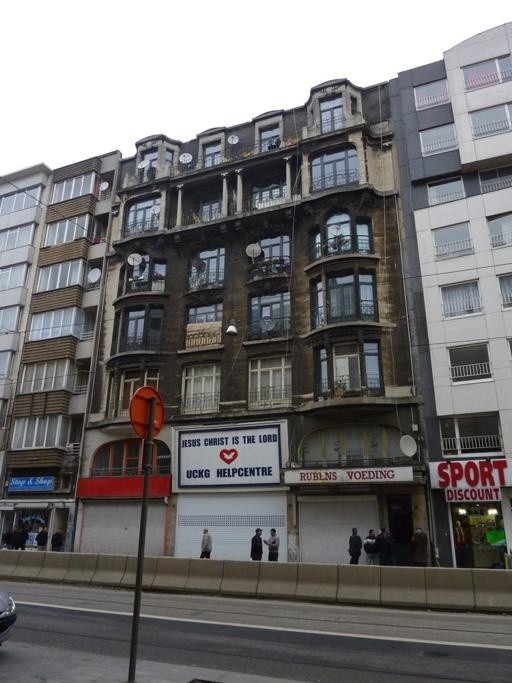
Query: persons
2 521 65 552
199 528 213 558
454 520 469 546
251 528 280 561
349 527 426 566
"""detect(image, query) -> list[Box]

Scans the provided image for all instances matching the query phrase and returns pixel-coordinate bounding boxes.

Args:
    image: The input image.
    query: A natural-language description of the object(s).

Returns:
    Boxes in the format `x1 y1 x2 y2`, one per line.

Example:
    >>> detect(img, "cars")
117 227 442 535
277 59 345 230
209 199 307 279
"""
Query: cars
0 582 16 651
